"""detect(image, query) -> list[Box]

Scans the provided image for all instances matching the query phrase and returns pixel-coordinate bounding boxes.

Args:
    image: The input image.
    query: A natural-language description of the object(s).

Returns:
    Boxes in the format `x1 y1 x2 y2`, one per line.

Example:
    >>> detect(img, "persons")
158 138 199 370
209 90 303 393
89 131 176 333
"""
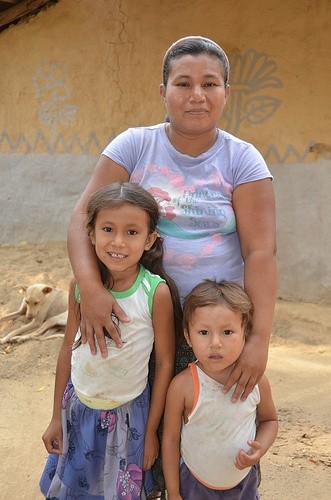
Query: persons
65 34 279 500
162 278 279 499
37 182 176 499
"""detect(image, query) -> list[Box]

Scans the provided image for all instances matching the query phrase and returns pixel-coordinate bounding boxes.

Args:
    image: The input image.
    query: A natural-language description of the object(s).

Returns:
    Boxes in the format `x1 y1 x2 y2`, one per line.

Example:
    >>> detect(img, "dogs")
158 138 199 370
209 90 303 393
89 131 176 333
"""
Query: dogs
0 284 69 345
